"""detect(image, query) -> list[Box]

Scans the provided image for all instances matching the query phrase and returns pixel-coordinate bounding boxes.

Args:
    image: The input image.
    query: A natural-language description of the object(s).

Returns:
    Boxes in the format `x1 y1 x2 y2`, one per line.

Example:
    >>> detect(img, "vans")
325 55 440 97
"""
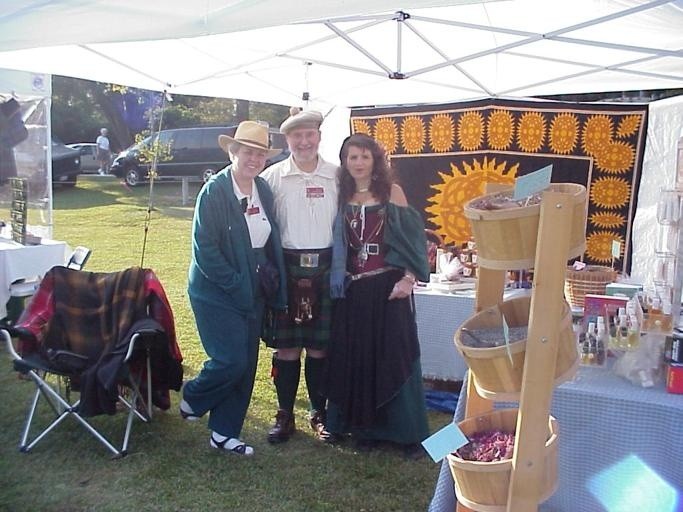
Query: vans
110 125 287 185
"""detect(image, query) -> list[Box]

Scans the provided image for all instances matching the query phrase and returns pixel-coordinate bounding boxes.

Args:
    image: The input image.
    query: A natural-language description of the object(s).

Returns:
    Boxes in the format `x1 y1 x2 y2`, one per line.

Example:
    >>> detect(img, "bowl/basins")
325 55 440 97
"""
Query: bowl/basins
462 181 588 270
453 294 578 403
446 408 561 511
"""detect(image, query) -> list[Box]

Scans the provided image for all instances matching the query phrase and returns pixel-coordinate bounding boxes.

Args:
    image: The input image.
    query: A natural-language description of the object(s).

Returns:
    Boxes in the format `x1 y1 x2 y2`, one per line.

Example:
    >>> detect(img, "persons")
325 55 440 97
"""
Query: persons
94 126 110 177
260 111 344 444
319 133 436 446
177 115 285 458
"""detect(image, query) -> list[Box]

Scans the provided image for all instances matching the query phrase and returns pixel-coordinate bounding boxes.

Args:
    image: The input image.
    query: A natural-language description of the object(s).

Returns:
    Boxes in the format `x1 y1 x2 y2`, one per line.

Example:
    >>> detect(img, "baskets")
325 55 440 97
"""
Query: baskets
564 261 619 312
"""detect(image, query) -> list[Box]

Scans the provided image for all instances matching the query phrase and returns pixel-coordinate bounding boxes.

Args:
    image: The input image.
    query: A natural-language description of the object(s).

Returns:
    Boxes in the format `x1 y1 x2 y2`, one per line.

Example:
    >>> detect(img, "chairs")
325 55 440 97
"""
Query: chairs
9 246 91 299
0 266 161 459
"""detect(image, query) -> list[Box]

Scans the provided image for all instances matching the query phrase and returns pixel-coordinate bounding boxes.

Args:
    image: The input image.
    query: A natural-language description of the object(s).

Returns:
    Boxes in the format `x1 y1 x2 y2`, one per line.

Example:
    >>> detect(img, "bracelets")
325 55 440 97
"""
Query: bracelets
403 276 416 285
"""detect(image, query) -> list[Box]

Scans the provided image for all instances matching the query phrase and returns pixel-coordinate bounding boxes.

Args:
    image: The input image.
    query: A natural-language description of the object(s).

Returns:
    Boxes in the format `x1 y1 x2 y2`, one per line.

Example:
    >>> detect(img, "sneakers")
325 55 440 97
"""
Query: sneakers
267 408 296 444
308 409 339 444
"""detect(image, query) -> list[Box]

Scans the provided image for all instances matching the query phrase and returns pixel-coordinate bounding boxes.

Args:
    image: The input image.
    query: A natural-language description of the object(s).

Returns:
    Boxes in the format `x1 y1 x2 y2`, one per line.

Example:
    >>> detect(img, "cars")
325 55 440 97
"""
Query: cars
66 142 118 176
50 136 79 187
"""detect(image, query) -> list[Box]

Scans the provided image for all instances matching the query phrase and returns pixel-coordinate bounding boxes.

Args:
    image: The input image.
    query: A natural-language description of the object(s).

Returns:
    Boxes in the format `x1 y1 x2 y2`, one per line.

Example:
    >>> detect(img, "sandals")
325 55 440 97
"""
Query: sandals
208 431 256 458
178 385 201 423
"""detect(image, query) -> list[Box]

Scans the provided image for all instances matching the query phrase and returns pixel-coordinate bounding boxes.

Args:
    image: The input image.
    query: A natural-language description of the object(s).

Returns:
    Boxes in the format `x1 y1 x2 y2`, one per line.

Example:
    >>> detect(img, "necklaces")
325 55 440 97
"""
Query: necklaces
351 186 370 195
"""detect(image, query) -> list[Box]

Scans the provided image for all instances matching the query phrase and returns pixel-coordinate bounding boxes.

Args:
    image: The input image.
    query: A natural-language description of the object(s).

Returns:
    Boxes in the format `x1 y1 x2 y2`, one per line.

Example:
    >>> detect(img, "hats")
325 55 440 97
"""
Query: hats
217 120 284 160
279 105 324 135
